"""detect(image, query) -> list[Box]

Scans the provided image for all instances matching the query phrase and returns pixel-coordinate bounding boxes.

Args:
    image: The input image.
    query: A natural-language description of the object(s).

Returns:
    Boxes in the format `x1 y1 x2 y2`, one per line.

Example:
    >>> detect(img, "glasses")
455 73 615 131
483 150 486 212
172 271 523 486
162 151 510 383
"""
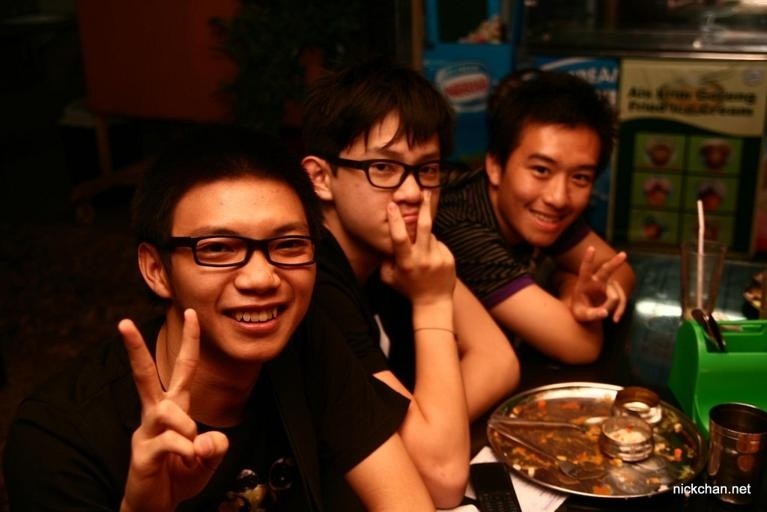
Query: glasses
325 156 456 189
167 235 319 268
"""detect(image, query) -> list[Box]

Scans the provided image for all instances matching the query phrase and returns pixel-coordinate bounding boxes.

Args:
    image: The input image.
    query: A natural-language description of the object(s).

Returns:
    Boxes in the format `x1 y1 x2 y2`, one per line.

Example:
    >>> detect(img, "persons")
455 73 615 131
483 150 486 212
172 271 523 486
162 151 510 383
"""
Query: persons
7 143 436 511
429 67 637 368
287 62 522 510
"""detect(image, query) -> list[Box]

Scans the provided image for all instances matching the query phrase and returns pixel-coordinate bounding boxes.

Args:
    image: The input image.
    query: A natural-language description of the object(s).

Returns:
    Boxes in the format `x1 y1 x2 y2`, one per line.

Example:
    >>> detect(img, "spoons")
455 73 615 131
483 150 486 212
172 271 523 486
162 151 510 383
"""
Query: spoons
487 415 612 436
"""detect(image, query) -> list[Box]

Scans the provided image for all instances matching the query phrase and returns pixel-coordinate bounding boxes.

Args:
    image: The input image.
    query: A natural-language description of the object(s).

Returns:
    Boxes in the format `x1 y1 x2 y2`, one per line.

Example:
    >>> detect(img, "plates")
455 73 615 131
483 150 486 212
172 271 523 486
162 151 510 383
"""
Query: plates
485 381 708 500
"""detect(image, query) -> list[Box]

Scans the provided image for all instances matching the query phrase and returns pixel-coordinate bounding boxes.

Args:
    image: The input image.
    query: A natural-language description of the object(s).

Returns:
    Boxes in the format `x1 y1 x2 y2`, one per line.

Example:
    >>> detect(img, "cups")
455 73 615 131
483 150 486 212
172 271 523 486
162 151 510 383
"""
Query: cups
679 237 728 320
704 402 767 506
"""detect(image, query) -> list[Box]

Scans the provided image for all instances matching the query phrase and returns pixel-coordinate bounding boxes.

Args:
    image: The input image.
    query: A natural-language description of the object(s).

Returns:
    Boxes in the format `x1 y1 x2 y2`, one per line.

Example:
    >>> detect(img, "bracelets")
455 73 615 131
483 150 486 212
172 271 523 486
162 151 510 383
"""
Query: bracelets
410 325 456 336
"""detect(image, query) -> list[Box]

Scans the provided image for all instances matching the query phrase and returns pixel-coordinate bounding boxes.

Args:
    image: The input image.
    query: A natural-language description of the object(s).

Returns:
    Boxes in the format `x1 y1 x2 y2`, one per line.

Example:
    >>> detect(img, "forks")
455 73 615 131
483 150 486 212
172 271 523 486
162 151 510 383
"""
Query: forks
487 420 610 482
